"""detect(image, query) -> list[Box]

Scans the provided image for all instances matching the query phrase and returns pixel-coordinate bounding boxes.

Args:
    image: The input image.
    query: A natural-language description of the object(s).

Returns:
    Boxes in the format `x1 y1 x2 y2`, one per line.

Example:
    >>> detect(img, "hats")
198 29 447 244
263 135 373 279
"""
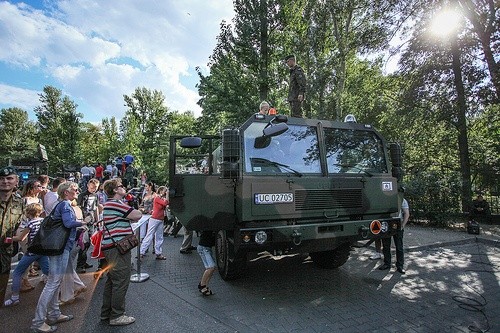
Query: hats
0 165 18 176
285 55 294 60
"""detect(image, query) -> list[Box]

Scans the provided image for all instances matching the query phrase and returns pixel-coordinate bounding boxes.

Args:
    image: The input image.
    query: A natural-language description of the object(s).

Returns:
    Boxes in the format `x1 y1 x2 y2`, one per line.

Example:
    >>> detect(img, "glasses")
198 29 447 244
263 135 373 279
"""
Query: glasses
71 189 77 192
116 184 125 188
34 184 42 187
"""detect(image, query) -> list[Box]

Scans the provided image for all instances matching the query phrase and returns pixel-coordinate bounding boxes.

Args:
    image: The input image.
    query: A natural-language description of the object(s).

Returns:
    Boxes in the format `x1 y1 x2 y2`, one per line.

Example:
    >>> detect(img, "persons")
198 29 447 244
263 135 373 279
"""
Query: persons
5 203 50 306
15 156 209 291
100 179 142 326
30 181 92 333
378 198 410 274
344 114 356 123
285 55 307 118
258 102 270 116
369 236 385 261
139 186 170 261
0 167 27 307
197 229 216 296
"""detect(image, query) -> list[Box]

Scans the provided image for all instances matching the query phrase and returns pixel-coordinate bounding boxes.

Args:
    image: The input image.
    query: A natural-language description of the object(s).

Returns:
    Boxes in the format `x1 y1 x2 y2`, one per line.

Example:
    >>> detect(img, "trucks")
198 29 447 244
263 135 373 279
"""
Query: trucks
168 108 407 282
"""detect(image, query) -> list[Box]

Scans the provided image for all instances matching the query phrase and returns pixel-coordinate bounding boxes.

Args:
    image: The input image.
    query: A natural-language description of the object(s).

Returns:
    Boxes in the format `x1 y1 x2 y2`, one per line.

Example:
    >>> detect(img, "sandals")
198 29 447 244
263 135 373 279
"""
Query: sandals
198 283 214 296
139 254 144 262
155 254 167 259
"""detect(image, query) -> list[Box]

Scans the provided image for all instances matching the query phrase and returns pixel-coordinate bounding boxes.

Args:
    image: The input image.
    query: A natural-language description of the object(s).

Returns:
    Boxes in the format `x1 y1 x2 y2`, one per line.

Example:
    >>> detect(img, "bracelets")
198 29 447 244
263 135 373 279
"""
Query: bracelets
401 225 404 228
84 221 88 224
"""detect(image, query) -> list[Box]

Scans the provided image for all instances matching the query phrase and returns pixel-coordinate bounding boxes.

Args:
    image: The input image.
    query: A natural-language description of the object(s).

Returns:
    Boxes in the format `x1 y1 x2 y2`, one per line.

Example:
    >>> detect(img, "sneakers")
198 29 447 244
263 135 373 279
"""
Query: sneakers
101 312 126 319
109 315 136 325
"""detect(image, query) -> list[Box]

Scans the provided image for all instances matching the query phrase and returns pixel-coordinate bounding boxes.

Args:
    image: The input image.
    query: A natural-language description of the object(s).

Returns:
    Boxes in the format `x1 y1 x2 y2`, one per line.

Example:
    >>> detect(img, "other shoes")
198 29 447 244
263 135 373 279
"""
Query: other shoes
179 246 198 254
28 268 39 276
380 263 391 270
4 298 19 306
93 268 104 279
20 281 36 292
30 264 41 270
76 266 86 273
29 273 87 333
397 266 405 274
376 249 383 252
163 231 178 238
84 262 94 269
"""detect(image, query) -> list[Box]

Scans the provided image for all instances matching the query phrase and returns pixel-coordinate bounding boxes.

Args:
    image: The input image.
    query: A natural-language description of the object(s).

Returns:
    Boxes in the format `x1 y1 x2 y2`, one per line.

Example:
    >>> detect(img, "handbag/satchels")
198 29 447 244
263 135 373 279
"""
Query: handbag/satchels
26 200 75 257
114 235 139 254
90 230 104 259
